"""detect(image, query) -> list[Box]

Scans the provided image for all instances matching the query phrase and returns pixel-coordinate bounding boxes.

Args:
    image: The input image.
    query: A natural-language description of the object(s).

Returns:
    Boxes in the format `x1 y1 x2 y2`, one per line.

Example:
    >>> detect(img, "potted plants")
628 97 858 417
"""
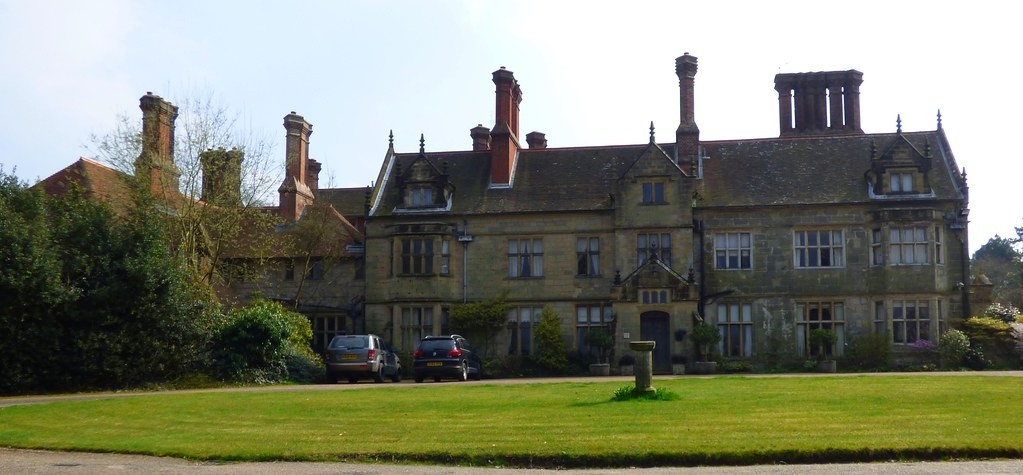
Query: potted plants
672 354 687 374
689 322 721 374
584 328 615 376
809 328 837 372
620 355 635 376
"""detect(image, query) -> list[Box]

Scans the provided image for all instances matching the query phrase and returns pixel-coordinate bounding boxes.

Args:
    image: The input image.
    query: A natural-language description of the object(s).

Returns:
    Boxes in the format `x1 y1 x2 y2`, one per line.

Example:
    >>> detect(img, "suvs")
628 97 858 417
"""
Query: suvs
411 333 483 383
325 334 402 383
